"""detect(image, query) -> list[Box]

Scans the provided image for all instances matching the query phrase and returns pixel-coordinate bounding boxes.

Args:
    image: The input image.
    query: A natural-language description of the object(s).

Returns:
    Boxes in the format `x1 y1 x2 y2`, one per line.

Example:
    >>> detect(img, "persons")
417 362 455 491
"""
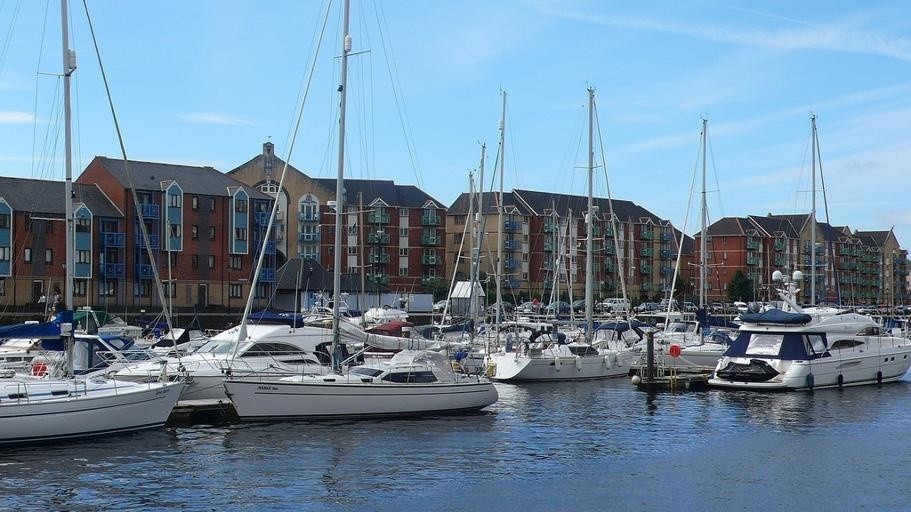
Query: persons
36 287 63 314
